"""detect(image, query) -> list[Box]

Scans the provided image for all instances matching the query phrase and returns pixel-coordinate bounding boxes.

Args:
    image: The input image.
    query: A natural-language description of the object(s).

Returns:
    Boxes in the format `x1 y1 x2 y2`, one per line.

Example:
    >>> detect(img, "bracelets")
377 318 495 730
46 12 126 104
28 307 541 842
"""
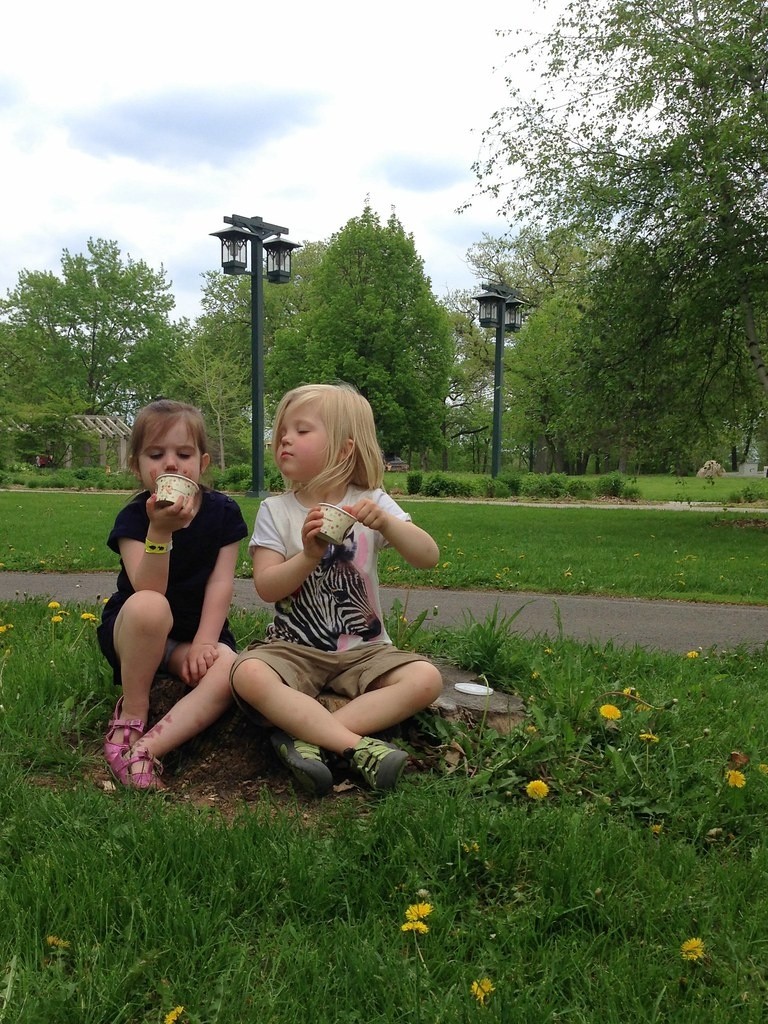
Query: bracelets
145 537 173 553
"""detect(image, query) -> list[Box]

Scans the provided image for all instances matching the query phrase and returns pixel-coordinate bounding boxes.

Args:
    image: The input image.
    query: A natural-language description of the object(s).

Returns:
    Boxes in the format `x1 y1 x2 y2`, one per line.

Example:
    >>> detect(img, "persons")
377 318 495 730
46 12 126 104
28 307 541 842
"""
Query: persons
36 453 54 469
229 383 442 796
97 399 248 792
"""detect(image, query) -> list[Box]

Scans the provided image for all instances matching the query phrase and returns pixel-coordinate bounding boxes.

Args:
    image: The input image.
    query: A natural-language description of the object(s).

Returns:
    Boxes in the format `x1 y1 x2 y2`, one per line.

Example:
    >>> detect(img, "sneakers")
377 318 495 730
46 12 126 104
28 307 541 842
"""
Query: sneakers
337 736 409 800
270 729 332 798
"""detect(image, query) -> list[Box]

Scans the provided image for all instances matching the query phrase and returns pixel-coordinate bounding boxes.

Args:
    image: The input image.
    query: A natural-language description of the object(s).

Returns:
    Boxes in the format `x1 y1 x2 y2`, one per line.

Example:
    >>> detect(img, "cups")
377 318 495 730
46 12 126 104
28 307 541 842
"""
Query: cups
317 502 358 545
155 474 199 508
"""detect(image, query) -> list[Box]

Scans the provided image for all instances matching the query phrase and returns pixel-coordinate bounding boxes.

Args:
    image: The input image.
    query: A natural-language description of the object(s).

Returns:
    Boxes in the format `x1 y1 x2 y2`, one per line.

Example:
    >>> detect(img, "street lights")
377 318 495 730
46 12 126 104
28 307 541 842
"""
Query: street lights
471 283 535 479
208 215 303 499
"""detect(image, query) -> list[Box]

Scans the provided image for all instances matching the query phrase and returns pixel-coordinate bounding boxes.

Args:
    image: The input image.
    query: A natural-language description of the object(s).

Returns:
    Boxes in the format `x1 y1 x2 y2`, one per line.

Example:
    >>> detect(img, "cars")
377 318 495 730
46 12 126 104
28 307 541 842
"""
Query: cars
386 461 407 472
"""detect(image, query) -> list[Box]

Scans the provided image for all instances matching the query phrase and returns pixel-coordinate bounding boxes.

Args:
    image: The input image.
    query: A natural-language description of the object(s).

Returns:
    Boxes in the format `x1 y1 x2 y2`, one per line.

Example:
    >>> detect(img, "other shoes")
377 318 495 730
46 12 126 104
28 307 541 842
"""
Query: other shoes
108 746 172 798
104 695 148 765
